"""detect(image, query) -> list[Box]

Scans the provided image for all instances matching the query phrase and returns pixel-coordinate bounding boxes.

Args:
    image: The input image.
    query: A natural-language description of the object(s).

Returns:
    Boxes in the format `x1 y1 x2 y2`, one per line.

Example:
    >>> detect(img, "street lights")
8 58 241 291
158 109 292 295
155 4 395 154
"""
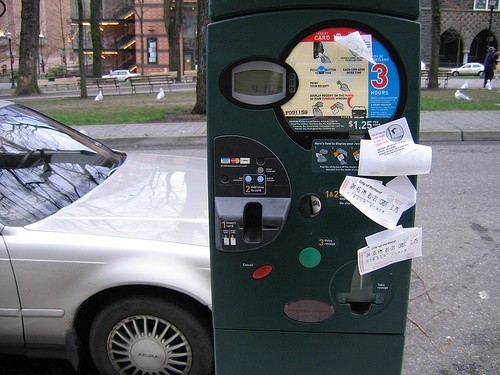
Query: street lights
39 32 44 75
485 0 497 53
6 32 17 89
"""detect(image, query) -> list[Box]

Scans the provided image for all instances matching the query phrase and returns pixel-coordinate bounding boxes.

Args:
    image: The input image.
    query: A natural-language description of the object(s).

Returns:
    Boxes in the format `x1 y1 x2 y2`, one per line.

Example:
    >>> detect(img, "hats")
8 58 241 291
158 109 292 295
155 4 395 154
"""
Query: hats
487 47 495 51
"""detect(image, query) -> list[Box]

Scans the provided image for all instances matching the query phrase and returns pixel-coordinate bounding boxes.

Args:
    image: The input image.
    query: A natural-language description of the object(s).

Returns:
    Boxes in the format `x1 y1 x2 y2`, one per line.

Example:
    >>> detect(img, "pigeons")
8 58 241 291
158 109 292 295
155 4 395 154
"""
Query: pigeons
460 80 468 89
94 91 103 101
156 87 165 100
454 90 469 100
485 79 492 90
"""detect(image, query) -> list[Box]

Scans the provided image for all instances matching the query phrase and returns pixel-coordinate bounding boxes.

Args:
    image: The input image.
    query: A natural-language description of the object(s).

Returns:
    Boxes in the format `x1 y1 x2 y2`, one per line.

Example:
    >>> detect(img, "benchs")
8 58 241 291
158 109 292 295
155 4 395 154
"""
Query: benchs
129 74 174 94
421 68 452 88
77 77 121 94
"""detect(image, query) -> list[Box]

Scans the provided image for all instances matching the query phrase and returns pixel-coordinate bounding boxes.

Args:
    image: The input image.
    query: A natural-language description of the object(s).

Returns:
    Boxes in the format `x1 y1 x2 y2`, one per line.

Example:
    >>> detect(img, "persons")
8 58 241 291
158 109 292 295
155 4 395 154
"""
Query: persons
483 47 498 88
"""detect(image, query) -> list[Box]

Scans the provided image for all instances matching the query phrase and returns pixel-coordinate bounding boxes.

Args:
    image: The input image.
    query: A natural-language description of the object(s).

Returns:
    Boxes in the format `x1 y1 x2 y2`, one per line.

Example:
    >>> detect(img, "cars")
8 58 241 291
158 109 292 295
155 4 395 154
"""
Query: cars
102 70 140 82
0 98 215 375
451 63 485 78
